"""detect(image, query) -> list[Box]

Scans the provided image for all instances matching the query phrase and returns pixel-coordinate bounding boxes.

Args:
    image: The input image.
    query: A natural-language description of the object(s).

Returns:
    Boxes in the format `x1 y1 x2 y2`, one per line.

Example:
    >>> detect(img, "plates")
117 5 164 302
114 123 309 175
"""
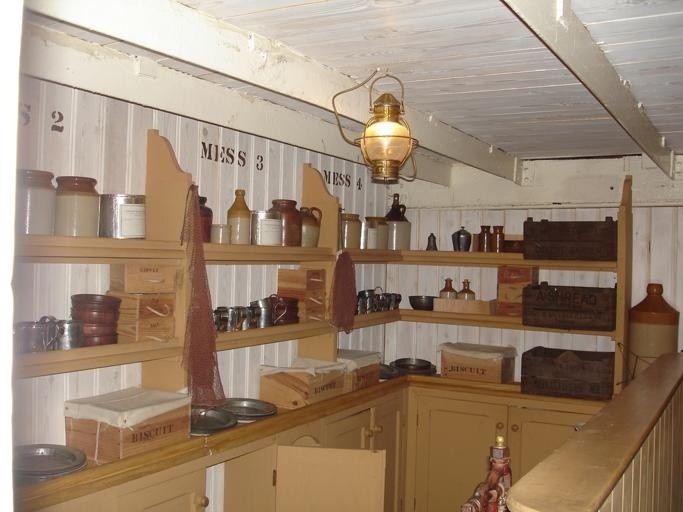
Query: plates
190 397 278 436
10 444 87 483
378 356 437 380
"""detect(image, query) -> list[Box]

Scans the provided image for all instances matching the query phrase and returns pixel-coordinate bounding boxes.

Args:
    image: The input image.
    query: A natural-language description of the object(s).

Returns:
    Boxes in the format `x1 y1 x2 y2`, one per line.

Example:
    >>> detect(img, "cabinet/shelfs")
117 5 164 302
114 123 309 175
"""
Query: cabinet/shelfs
14 129 683 511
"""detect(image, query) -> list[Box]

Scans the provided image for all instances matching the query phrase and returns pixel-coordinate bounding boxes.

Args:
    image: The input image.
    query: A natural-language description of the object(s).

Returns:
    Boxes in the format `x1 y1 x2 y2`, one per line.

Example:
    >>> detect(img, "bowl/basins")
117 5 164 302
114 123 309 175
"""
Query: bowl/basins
270 296 301 326
70 293 122 348
408 295 437 311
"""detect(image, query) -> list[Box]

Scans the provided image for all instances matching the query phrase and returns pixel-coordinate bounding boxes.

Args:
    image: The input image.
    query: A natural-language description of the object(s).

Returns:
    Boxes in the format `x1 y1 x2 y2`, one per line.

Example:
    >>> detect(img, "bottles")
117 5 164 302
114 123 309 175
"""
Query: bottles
14 169 505 254
439 277 476 302
629 283 679 381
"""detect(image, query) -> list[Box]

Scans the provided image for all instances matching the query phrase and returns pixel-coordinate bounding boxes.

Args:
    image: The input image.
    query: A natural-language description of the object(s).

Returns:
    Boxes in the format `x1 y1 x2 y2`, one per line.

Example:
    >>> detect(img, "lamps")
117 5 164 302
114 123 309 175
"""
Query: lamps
332 67 419 186
